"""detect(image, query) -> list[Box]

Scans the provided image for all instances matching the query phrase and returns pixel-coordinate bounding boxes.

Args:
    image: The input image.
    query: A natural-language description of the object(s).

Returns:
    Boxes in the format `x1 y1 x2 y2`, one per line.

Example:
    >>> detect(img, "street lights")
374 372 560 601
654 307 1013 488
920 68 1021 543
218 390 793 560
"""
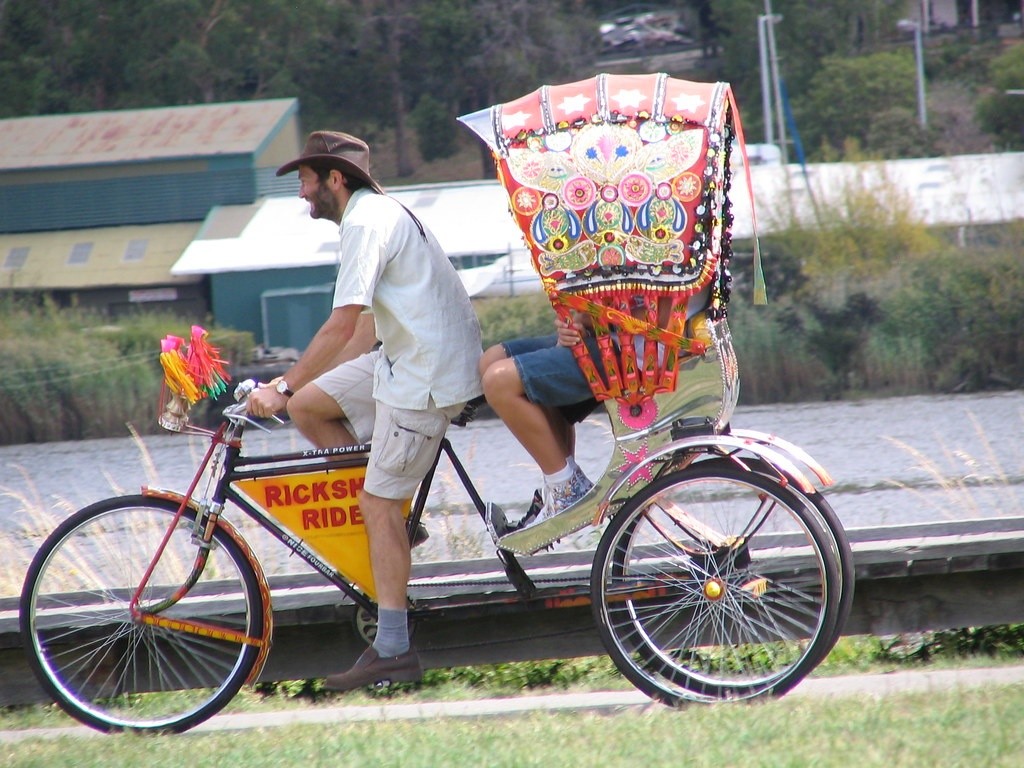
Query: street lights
755 11 788 147
895 16 930 128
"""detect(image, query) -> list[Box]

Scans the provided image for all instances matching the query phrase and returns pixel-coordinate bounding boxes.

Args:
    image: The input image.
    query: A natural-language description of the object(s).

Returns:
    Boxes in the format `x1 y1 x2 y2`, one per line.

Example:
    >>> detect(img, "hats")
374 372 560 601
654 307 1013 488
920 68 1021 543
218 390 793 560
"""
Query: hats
277 130 385 197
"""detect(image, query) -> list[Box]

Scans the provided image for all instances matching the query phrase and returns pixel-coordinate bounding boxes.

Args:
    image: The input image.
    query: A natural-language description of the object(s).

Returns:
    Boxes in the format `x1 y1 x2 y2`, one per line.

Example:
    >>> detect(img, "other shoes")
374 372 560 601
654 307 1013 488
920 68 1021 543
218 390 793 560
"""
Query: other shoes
518 467 597 530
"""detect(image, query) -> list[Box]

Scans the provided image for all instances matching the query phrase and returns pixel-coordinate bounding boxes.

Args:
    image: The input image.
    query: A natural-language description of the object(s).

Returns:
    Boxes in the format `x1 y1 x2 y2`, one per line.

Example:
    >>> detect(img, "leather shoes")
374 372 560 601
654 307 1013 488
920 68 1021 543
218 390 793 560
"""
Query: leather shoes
403 515 428 549
325 643 422 690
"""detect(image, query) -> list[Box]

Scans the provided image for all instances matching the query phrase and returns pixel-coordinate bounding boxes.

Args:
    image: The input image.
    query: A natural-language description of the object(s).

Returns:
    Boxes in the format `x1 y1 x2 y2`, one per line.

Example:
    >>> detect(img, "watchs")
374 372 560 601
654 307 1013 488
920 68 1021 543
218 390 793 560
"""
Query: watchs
275 380 295 398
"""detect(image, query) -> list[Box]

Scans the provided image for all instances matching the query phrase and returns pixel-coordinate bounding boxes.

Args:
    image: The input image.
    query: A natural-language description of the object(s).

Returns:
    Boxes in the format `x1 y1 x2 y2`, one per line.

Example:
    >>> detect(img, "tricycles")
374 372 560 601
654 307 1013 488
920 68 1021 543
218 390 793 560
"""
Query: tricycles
19 375 856 739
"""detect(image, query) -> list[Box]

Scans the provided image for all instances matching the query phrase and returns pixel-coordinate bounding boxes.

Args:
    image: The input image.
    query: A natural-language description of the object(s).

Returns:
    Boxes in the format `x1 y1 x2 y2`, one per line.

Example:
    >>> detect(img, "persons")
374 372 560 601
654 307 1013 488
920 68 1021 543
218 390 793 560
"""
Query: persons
479 281 711 536
244 130 485 691
